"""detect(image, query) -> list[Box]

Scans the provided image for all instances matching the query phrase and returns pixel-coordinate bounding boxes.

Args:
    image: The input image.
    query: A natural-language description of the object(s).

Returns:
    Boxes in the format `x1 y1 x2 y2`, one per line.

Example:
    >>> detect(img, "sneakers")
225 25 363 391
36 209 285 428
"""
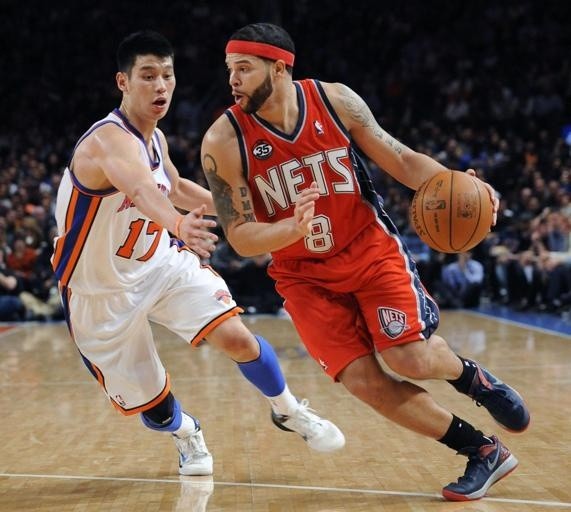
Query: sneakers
451 357 530 433
271 396 345 450
171 427 213 476
441 435 518 501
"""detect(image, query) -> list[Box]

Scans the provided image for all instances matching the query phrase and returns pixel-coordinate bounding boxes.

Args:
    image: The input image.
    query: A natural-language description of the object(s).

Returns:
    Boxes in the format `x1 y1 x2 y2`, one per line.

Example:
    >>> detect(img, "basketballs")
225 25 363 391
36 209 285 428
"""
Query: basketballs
410 169 494 254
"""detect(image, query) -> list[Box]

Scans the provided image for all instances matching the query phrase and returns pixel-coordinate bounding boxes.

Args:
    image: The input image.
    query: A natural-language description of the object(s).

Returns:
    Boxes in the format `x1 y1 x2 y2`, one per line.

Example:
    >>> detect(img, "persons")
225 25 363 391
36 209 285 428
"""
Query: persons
51 31 346 475
174 475 214 512
1 125 570 319
201 23 531 501
